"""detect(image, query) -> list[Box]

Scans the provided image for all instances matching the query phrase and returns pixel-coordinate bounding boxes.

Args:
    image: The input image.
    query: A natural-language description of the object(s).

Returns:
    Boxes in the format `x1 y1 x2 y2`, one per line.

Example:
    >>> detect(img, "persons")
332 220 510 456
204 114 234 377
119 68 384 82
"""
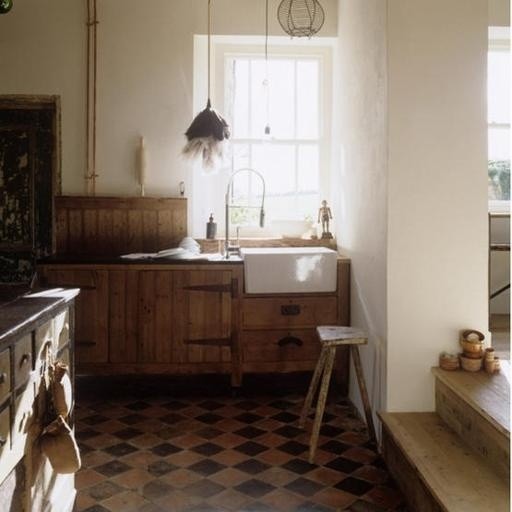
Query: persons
318 200 333 240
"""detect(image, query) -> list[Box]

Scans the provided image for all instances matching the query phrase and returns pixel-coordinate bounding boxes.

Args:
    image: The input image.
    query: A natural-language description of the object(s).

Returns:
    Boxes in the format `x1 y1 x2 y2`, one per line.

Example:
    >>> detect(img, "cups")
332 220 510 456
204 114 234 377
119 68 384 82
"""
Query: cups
486 347 501 374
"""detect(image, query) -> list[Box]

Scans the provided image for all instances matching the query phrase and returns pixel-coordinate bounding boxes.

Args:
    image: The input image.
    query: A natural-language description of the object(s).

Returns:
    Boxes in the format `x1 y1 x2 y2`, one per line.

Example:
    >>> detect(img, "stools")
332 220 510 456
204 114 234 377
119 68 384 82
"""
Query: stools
298 325 379 465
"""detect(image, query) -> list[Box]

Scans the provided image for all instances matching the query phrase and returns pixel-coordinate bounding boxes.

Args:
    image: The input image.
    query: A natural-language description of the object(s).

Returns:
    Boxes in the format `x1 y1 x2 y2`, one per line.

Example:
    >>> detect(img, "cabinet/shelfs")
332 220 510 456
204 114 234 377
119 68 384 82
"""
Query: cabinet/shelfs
1 286 81 512
1 259 350 398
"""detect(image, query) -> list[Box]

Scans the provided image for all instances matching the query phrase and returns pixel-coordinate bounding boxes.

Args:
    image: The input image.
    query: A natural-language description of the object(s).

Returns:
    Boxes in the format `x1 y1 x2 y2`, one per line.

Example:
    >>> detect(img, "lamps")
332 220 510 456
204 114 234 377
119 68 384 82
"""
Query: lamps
276 0 326 37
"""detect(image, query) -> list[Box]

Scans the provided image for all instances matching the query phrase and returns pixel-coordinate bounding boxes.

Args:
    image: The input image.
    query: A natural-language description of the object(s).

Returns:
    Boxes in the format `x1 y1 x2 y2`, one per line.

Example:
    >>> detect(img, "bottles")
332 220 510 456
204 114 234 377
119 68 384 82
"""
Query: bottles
205 212 218 239
179 181 185 196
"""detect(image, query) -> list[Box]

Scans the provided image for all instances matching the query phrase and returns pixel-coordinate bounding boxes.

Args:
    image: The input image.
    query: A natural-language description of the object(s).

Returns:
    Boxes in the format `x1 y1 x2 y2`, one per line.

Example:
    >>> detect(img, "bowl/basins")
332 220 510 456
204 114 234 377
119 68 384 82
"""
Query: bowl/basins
459 328 485 352
460 352 482 373
438 353 458 371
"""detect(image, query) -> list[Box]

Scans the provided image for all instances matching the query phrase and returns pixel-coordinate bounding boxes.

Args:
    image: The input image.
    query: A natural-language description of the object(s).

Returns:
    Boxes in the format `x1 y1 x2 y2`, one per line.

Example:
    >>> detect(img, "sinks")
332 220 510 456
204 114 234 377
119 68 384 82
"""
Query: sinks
240 246 338 294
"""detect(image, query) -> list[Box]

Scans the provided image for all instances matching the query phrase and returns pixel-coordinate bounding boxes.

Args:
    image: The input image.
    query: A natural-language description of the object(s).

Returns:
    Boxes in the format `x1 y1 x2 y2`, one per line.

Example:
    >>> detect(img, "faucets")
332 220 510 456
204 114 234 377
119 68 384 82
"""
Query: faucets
224 167 266 258
